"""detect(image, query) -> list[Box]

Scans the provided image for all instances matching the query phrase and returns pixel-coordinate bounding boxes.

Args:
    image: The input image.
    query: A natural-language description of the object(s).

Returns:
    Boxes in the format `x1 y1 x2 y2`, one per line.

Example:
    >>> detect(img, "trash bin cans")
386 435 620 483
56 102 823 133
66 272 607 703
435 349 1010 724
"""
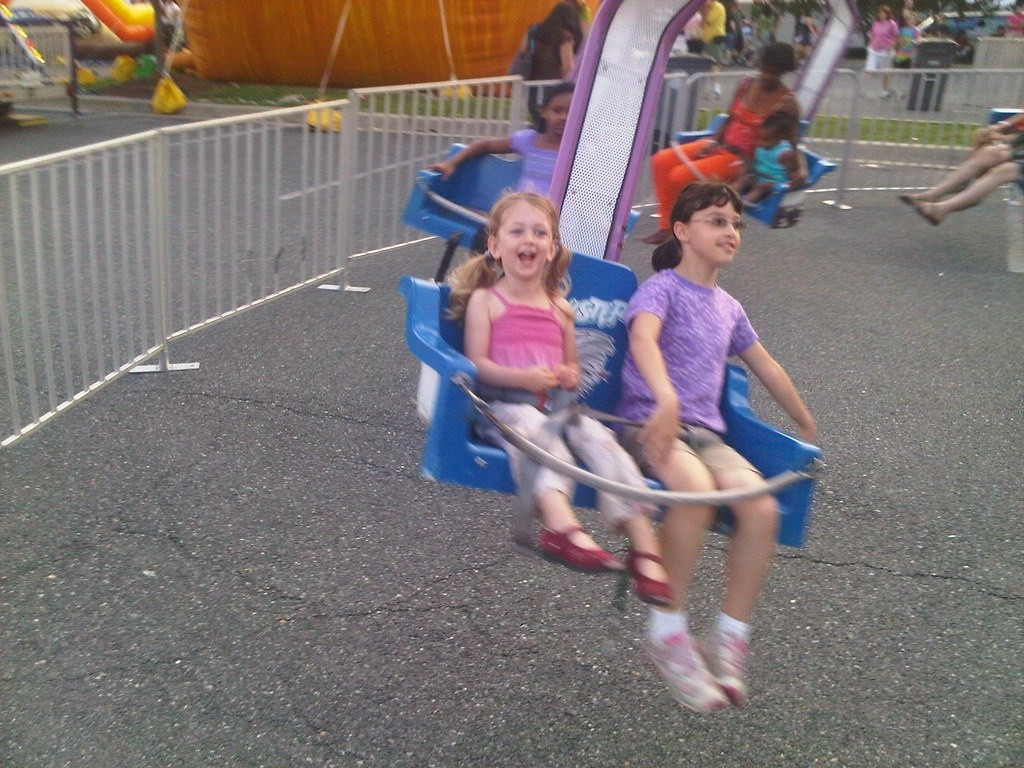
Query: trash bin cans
653 55 717 155
906 38 961 112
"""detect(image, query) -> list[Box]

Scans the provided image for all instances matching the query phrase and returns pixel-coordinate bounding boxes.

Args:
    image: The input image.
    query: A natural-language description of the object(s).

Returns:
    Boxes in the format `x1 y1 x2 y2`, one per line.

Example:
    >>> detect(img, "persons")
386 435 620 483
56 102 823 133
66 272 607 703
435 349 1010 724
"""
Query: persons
635 0 1024 244
427 82 573 199
465 189 681 610
518 0 587 122
617 180 809 713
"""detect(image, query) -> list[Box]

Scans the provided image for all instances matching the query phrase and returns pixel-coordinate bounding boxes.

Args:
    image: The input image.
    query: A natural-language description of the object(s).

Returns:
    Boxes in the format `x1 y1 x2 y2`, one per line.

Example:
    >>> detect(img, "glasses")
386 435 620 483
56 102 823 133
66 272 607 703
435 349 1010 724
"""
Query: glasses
686 215 750 231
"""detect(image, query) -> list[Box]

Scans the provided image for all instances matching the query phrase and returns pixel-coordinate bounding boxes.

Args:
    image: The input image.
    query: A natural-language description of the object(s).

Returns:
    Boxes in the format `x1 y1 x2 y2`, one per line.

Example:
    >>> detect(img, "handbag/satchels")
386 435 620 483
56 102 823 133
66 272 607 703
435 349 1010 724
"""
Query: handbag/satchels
507 23 537 92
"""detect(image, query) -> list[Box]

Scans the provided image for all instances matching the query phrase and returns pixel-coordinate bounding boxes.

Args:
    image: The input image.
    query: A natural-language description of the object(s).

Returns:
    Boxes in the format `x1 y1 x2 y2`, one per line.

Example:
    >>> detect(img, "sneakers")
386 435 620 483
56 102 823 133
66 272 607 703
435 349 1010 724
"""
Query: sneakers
648 632 731 712
701 627 749 704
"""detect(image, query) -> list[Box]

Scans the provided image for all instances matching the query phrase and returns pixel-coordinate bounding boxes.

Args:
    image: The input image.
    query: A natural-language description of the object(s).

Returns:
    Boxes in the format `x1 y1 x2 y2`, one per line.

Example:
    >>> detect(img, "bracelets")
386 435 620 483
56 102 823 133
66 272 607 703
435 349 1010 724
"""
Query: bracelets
997 120 1011 126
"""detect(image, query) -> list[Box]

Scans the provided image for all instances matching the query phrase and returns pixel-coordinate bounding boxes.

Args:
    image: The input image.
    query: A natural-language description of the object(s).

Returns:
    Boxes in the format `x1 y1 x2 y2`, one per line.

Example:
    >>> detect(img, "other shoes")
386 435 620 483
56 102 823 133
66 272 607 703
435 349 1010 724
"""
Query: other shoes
537 524 627 573
880 90 894 99
645 229 674 244
628 544 674 608
713 82 721 96
899 193 917 206
911 201 944 226
